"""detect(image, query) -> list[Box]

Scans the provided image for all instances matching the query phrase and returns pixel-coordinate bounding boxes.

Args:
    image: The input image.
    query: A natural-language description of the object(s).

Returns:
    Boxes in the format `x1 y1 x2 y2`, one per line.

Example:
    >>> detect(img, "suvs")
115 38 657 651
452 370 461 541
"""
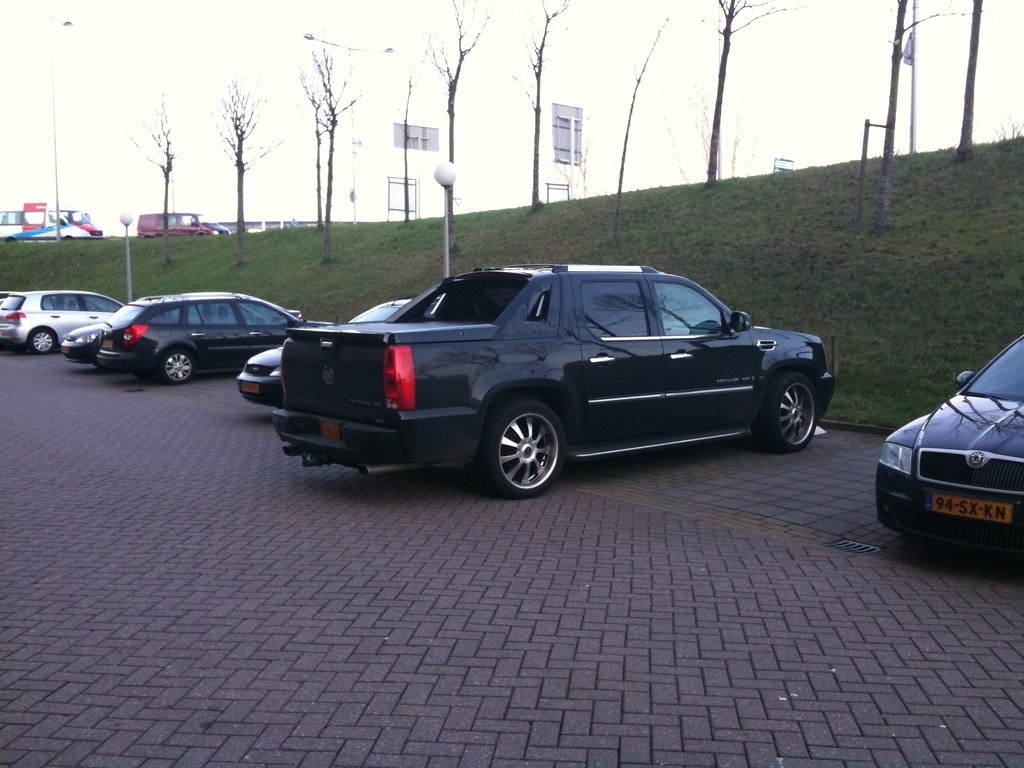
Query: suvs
60 292 334 386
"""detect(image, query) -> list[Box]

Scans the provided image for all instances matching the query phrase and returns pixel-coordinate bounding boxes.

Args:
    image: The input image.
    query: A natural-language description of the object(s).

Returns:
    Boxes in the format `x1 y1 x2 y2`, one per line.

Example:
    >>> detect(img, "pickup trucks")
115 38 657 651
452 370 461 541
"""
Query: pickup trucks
271 264 836 500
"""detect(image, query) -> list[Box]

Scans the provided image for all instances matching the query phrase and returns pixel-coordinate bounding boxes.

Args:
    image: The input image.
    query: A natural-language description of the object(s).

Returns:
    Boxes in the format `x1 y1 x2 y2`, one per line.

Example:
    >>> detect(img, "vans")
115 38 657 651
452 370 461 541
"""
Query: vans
136 213 230 239
0 203 103 243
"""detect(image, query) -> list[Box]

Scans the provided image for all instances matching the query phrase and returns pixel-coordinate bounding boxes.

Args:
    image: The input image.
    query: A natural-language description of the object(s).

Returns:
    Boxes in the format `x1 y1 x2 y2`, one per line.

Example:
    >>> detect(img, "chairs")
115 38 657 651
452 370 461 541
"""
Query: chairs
15 214 29 224
67 300 77 310
218 306 228 318
2 216 7 224
56 299 65 309
49 215 56 223
201 304 213 317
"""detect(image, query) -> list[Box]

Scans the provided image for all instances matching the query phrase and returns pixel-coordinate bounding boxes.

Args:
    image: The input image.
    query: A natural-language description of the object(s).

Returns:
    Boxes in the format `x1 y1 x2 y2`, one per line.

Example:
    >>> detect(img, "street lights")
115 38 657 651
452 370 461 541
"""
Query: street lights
46 18 74 244
304 33 395 224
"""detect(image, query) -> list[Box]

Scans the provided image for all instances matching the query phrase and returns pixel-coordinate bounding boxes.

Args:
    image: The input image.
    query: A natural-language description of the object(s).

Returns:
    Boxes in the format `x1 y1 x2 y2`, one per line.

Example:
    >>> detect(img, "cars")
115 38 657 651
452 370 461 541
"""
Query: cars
237 295 442 409
876 334 1024 564
0 291 125 355
